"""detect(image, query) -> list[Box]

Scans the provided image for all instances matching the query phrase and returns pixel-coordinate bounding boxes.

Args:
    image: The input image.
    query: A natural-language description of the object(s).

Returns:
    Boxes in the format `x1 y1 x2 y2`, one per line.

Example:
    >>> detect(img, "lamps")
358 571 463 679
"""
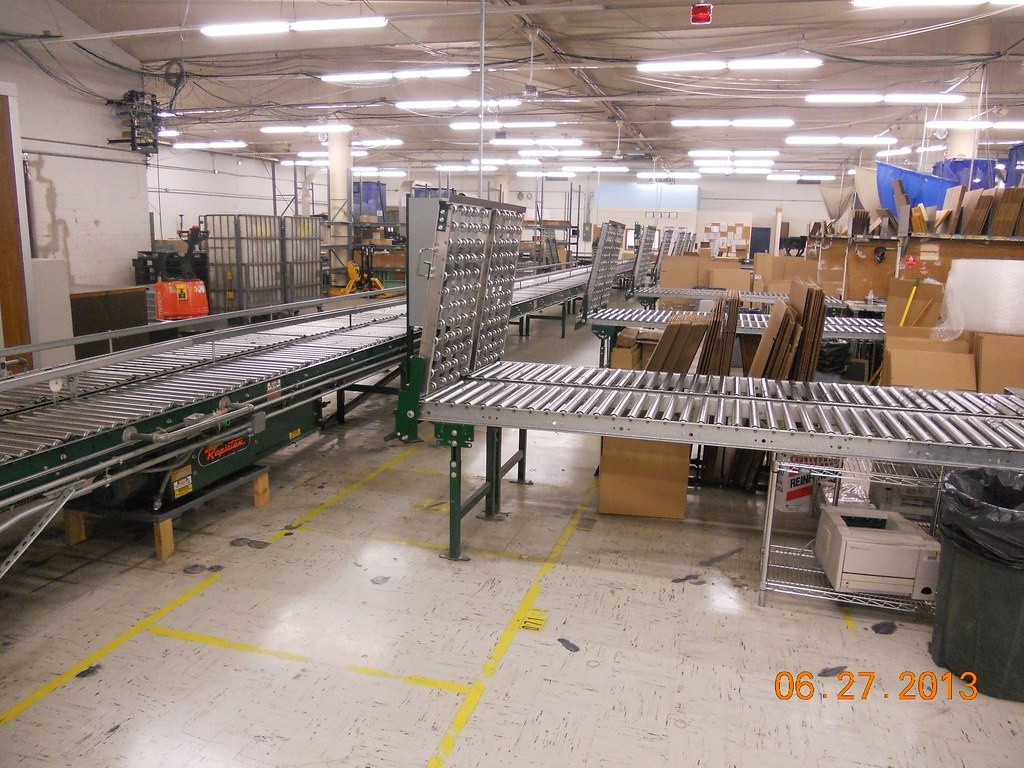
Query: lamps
155 0 1024 182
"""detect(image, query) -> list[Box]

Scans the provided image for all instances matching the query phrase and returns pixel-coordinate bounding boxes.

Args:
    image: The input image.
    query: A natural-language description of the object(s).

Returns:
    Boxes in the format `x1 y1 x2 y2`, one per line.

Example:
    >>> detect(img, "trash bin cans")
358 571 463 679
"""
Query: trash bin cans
928 467 1024 703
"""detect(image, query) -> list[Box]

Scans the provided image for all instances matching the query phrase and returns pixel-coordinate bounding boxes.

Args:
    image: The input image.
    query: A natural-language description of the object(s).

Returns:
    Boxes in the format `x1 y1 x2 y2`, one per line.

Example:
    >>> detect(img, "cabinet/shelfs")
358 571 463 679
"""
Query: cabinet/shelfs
755 447 945 615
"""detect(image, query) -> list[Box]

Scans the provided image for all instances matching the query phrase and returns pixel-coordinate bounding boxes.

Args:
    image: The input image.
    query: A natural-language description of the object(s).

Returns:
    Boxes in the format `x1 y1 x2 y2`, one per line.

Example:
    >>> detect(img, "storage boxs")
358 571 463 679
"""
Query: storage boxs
774 452 839 513
610 328 664 372
882 277 1024 394
657 253 819 315
597 436 691 522
